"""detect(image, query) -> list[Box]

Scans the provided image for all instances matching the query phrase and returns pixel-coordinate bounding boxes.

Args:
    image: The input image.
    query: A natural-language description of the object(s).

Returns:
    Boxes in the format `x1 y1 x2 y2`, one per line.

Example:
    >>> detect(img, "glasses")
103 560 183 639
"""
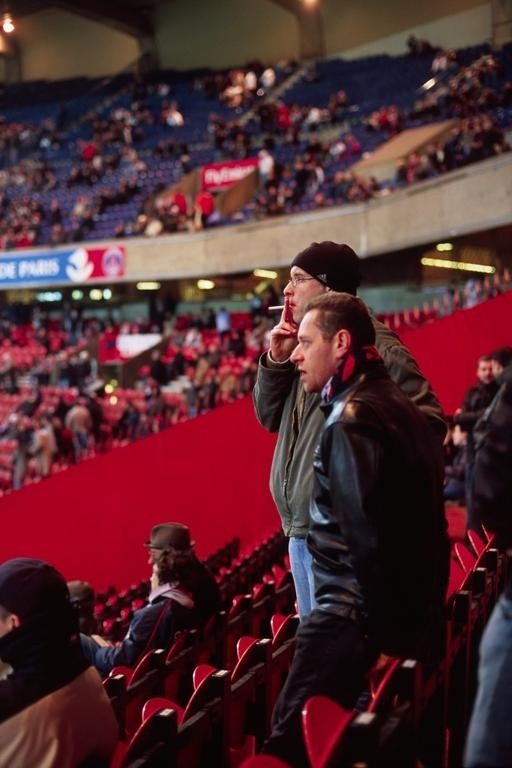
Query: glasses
291 276 316 287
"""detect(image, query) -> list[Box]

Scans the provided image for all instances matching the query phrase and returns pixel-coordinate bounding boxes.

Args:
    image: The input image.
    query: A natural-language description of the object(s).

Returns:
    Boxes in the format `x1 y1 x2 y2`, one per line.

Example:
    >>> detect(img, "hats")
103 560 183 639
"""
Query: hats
289 239 361 296
0 557 69 617
143 521 196 549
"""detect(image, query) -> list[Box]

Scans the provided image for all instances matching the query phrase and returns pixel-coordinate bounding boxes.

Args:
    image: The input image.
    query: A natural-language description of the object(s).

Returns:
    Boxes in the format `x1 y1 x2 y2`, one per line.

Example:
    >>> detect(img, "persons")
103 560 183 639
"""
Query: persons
0 555 118 768
0 303 284 490
0 34 511 250
452 353 499 450
443 417 471 502
251 239 446 622
95 549 213 677
471 346 512 531
261 290 453 768
146 521 225 631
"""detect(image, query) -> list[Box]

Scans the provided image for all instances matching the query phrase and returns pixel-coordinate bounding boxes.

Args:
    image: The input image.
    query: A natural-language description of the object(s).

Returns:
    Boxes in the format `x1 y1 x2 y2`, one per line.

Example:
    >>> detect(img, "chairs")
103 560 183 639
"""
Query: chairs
141 669 222 766
264 554 292 605
260 521 290 573
73 576 161 650
165 631 191 698
467 528 490 567
236 613 291 682
114 708 186 768
250 582 271 639
206 610 225 673
363 656 417 759
190 640 258 714
199 525 260 601
270 597 300 672
482 520 499 550
93 674 126 710
227 595 252 649
110 649 162 702
301 696 375 768
